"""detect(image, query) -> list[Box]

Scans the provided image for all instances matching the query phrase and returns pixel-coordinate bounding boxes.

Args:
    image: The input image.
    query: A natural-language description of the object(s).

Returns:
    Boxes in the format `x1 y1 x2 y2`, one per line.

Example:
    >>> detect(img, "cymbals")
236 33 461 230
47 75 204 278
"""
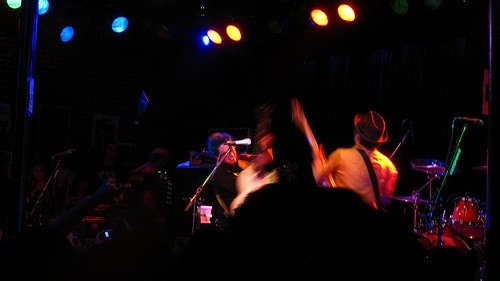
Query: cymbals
473 166 486 170
393 196 429 204
412 165 446 175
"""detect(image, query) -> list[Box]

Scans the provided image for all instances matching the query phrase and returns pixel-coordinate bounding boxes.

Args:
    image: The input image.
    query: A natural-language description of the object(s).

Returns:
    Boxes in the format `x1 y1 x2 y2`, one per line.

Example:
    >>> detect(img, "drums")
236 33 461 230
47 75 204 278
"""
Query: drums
450 197 480 225
421 231 471 250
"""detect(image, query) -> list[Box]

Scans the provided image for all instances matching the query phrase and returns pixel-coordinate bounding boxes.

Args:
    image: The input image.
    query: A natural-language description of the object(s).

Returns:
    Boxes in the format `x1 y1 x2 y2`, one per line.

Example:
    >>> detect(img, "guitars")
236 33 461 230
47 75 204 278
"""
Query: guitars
291 98 336 187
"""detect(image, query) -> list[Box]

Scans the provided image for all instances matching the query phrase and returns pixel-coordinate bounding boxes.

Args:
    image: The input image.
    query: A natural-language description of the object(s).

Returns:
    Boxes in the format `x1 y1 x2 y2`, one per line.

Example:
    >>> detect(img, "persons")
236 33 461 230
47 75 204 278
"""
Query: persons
0 183 483 281
23 140 186 246
207 132 276 217
312 111 398 209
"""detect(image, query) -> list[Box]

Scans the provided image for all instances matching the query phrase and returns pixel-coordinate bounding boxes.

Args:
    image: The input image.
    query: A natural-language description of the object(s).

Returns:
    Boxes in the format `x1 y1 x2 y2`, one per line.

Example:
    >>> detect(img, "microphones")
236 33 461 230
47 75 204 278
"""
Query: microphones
226 138 252 146
53 148 77 159
458 117 483 126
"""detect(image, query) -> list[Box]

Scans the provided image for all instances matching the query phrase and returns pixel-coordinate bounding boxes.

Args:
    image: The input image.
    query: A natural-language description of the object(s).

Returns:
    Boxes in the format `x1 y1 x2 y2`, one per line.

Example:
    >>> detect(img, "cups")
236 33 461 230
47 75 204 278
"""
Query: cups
190 151 196 163
200 206 212 223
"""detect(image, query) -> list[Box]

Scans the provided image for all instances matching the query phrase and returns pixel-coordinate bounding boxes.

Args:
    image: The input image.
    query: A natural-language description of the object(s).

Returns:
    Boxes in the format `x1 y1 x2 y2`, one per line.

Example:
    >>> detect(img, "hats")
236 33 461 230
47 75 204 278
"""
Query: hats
354 110 388 143
